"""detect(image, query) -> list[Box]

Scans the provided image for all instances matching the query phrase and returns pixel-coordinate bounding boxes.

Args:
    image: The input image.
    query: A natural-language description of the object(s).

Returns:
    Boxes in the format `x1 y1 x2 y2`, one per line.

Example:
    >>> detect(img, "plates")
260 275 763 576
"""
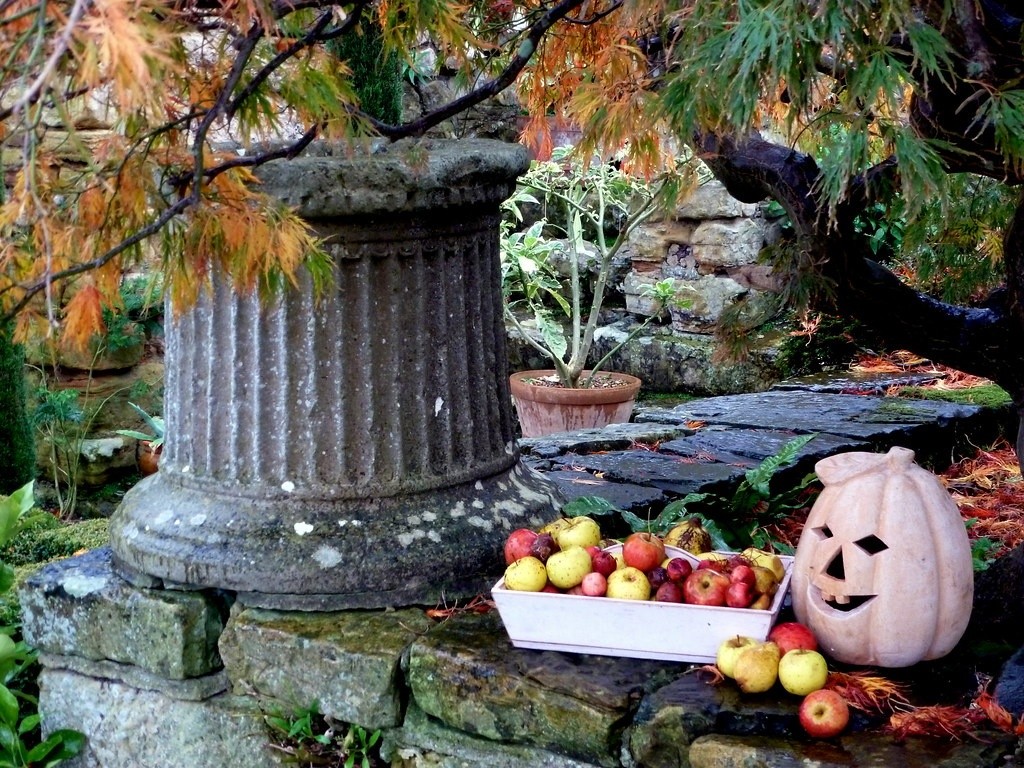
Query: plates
599 542 703 572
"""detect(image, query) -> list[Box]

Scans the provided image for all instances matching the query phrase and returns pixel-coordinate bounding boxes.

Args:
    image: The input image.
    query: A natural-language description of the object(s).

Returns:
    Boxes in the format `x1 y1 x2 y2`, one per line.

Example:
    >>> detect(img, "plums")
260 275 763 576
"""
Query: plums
582 572 606 595
730 566 755 585
586 546 601 559
530 534 559 563
725 582 752 608
593 552 616 575
667 558 692 581
647 567 667 588
656 583 682 602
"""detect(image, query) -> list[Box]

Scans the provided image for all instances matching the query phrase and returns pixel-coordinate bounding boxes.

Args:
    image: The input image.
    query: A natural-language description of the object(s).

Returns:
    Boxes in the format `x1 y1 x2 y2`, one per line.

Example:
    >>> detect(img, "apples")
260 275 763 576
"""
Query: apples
546 545 592 588
800 690 850 737
624 532 665 570
559 515 600 550
770 622 816 655
717 634 758 679
742 548 783 581
504 554 547 592
504 528 537 564
683 569 730 606
607 568 651 600
779 645 827 695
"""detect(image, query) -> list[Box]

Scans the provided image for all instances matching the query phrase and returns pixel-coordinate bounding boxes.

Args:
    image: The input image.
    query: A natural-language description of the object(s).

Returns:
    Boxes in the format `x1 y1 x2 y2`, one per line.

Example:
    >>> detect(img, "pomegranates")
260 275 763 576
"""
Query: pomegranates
665 518 711 557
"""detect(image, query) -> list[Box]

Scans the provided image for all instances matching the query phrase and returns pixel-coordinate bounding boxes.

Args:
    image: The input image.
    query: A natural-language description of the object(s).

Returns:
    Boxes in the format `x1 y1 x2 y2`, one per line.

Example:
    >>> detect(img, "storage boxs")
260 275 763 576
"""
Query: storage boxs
490 543 796 663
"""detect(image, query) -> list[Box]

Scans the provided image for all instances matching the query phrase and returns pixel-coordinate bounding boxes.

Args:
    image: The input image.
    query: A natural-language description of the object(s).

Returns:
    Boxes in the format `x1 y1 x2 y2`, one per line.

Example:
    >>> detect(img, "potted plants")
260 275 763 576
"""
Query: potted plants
497 132 714 440
116 401 163 475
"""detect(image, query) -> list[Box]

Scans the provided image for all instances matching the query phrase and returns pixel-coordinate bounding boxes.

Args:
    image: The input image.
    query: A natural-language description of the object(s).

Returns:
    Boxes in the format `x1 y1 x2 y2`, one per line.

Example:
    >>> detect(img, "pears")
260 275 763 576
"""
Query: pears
735 641 780 693
